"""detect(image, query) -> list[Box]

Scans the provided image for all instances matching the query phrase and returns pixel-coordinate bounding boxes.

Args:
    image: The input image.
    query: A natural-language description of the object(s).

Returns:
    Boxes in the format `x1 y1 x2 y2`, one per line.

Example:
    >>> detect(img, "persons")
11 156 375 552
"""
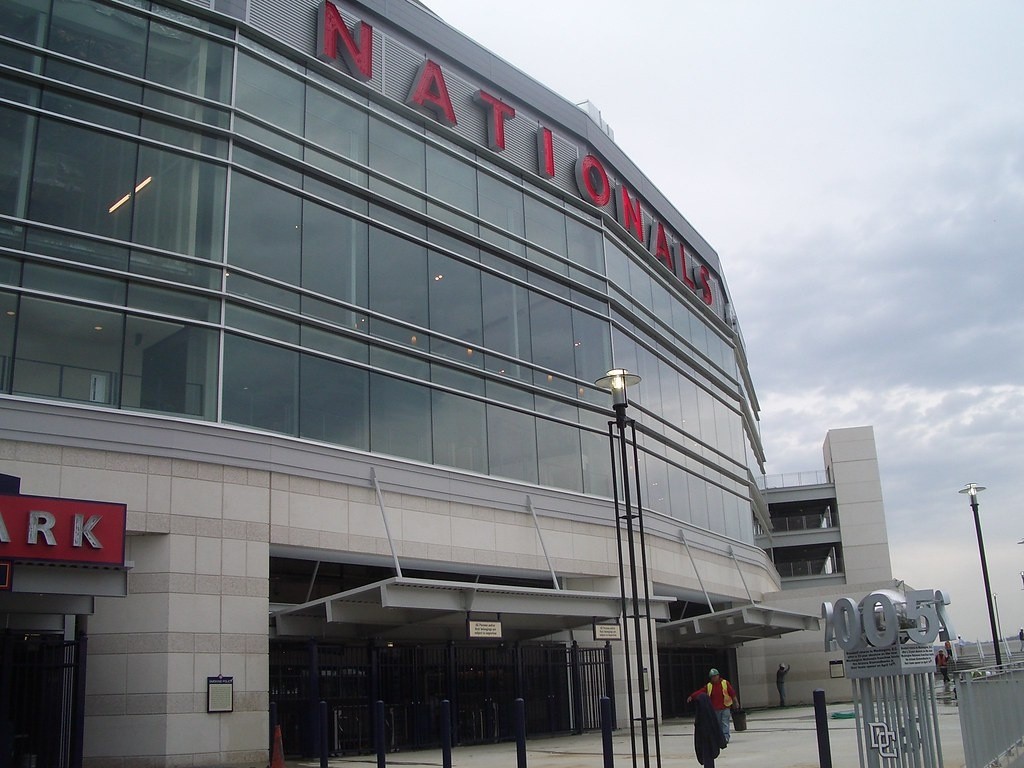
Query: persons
776 663 790 707
935 649 951 682
1019 628 1024 651
958 636 965 655
945 638 952 657
687 669 739 743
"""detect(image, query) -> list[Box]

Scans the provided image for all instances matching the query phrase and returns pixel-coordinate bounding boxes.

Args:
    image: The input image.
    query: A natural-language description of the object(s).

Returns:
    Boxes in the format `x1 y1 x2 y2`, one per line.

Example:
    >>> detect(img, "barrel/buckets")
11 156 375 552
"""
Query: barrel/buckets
732 710 746 731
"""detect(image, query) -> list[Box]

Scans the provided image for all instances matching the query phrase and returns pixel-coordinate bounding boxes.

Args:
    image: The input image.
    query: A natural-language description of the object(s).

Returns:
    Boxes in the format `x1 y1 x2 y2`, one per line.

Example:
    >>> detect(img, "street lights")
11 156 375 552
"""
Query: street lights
957 481 1003 675
992 593 1002 642
592 369 661 768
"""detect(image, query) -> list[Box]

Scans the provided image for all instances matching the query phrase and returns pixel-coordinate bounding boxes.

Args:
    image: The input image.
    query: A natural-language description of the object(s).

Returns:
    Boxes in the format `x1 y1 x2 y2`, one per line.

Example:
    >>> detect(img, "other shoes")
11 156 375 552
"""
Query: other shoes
726 737 729 742
780 704 787 707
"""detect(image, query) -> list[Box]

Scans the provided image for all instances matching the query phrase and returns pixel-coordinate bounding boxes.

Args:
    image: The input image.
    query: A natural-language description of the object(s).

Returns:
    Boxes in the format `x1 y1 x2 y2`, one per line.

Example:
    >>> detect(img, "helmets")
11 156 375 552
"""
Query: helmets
939 649 943 652
709 668 719 677
780 663 785 667
